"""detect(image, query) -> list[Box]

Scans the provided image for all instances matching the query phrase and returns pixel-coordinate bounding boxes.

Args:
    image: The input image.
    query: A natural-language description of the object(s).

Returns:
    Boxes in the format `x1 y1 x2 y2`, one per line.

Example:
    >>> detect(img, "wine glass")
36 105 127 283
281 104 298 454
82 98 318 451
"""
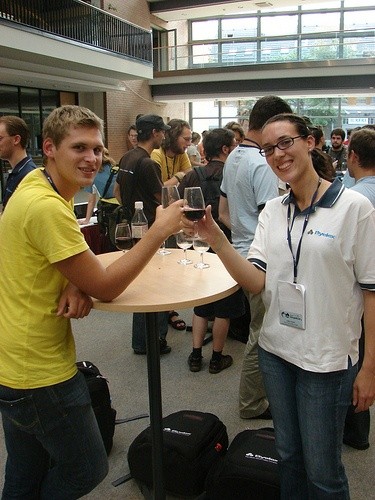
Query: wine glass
193 234 210 269
161 186 180 209
183 187 206 241
174 230 193 264
114 223 131 255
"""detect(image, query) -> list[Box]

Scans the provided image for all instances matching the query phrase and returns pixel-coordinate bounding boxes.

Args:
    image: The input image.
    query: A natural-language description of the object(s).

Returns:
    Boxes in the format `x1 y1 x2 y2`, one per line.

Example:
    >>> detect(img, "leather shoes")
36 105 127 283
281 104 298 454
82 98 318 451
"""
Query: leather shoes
251 406 272 420
342 435 369 450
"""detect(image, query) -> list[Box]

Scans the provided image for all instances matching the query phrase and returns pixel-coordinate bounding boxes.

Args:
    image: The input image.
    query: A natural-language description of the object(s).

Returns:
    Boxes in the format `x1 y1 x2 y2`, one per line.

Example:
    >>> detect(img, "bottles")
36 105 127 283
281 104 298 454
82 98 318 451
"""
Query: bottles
130 201 149 247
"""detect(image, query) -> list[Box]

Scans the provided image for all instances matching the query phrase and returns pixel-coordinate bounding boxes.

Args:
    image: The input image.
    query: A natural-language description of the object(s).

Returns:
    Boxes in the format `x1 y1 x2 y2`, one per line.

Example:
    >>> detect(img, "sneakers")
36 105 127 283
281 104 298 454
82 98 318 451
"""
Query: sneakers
187 351 203 371
159 337 167 347
133 346 171 354
209 354 233 373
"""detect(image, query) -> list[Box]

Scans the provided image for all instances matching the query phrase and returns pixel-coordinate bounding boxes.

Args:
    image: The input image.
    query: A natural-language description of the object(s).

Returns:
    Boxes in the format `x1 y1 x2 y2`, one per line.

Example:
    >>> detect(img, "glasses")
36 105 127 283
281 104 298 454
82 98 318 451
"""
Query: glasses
130 135 137 138
235 138 242 144
180 136 192 142
259 134 304 157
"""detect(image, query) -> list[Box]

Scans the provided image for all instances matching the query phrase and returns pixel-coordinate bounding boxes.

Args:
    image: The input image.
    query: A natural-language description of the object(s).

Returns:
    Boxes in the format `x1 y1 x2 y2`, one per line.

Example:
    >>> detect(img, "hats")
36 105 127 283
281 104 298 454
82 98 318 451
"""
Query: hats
136 115 171 130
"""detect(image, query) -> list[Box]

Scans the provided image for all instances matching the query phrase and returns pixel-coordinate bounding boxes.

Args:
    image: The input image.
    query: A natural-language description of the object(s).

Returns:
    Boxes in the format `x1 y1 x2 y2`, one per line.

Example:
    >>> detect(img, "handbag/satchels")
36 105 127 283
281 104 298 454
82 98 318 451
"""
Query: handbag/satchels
97 199 135 248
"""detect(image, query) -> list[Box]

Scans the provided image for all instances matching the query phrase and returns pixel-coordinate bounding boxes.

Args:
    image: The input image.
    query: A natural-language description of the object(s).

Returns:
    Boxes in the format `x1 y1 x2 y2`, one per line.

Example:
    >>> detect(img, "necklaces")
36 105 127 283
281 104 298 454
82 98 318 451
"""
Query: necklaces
42 168 61 196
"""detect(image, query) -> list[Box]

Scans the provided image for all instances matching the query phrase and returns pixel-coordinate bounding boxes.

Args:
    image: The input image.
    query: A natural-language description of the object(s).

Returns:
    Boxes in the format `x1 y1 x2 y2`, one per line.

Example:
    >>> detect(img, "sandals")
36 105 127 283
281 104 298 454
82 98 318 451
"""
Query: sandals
168 312 186 330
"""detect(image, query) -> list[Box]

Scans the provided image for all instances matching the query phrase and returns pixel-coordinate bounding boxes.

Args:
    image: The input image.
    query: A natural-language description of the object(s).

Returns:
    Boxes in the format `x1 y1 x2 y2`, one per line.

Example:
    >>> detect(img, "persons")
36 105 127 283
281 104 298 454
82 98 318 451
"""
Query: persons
200 129 209 138
325 128 347 171
308 126 339 182
216 95 293 420
223 121 246 150
134 113 144 121
0 115 38 217
77 147 122 252
177 112 375 499
167 127 240 374
127 124 138 150
185 131 208 169
347 127 375 210
113 114 171 356
0 105 187 500
344 129 354 145
300 116 313 126
151 120 193 331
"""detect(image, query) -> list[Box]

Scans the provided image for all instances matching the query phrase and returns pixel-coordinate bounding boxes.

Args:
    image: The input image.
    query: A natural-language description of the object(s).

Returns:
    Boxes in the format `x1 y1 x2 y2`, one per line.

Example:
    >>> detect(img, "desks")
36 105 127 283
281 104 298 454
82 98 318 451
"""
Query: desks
89 246 243 484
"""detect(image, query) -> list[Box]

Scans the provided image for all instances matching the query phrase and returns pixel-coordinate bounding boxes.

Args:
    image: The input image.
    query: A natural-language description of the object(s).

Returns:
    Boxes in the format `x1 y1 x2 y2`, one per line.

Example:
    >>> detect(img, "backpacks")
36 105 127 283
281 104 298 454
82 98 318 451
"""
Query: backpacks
112 410 228 500
75 361 149 456
192 167 231 240
206 427 281 500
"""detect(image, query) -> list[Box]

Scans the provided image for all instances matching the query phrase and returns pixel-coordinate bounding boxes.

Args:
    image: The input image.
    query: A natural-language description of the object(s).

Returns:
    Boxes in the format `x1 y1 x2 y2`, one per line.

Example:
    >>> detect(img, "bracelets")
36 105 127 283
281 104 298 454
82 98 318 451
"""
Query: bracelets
212 231 224 252
